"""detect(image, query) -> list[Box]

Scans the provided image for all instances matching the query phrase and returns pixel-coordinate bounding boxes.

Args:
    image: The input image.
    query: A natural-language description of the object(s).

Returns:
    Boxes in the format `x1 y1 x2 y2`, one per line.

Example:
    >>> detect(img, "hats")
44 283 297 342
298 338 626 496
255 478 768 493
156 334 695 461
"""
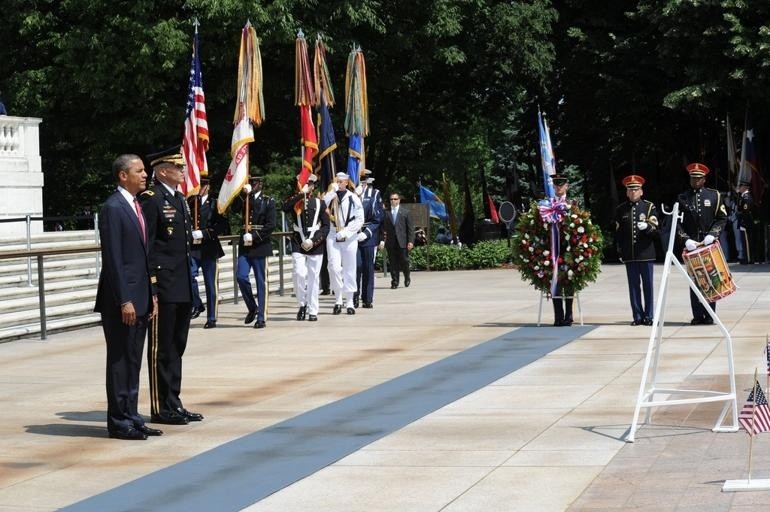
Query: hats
550 174 568 186
622 175 645 190
687 164 710 178
200 176 210 185
295 173 318 183
248 176 263 185
145 144 187 167
336 168 375 184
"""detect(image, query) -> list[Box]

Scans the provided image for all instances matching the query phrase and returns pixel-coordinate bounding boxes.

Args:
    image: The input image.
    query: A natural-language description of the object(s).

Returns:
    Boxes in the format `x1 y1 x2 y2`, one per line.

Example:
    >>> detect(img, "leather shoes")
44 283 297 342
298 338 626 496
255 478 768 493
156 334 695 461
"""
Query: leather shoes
109 408 203 440
245 310 257 324
191 304 205 319
405 279 410 287
645 319 653 326
309 315 317 321
392 285 397 289
254 320 266 328
297 306 306 321
333 295 373 315
320 289 330 295
632 320 645 326
203 321 216 328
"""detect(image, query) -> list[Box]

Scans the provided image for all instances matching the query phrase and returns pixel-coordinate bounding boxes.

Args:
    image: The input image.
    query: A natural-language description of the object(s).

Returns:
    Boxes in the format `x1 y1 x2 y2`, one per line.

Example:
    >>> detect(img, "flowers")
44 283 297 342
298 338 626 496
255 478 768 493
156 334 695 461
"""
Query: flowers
508 197 607 301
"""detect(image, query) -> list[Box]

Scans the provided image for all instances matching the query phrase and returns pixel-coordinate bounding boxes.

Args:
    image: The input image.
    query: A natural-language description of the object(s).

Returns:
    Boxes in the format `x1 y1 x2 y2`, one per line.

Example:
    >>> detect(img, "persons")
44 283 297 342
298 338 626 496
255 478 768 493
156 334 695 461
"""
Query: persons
609 175 659 325
413 225 428 246
356 168 387 308
547 172 575 327
140 170 157 202
434 224 454 245
281 173 332 321
654 177 765 266
140 142 204 425
231 172 278 328
187 176 225 328
93 153 164 440
382 191 417 289
675 161 729 325
321 171 366 314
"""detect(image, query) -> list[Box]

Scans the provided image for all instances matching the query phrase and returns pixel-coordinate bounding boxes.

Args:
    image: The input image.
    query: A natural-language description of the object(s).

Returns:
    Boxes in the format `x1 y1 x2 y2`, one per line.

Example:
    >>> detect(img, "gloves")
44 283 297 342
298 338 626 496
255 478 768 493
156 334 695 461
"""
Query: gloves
191 229 203 240
242 233 253 243
638 221 647 230
243 183 252 194
302 184 310 194
335 229 345 241
354 184 364 195
331 182 339 192
301 239 314 252
685 239 698 251
703 235 715 245
357 232 367 242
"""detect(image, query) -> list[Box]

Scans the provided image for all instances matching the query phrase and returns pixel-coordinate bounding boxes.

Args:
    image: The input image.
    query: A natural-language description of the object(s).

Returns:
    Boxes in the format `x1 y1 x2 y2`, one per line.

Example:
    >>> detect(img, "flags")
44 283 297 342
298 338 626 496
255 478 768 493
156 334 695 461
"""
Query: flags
738 381 770 437
537 112 559 199
544 122 556 166
489 196 501 225
766 343 770 377
215 27 266 217
726 117 739 178
420 186 450 221
312 38 339 192
737 107 761 201
343 51 371 192
176 33 210 201
294 36 320 188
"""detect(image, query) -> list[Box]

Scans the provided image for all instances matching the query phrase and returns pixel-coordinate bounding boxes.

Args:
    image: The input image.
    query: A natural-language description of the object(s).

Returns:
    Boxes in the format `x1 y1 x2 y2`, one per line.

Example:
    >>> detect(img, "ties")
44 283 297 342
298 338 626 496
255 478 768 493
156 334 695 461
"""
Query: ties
393 208 397 225
133 198 146 247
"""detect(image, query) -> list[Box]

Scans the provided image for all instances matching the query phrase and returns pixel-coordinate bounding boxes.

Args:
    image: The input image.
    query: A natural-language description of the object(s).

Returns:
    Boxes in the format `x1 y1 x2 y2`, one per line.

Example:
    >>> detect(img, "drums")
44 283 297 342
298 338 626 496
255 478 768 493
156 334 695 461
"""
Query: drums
682 240 738 303
